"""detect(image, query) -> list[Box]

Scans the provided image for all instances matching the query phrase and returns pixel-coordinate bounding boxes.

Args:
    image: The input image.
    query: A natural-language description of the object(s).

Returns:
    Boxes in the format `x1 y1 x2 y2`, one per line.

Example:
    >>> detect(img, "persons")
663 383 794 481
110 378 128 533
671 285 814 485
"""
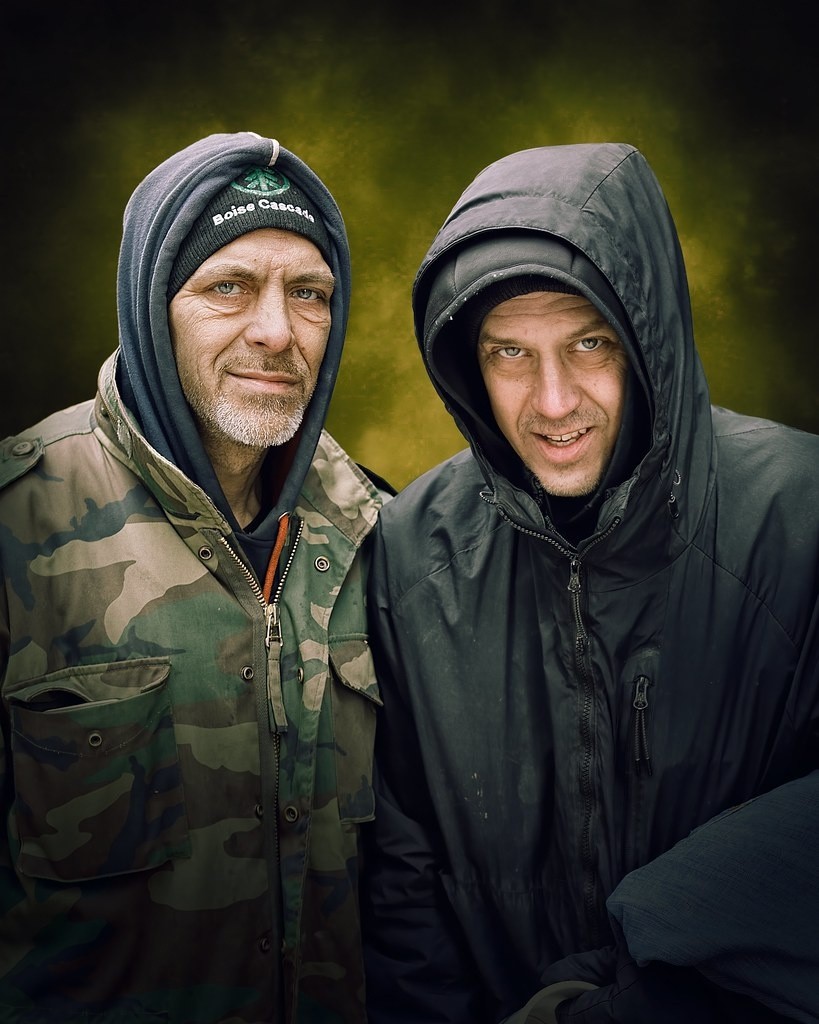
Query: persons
366 143 819 1024
0 131 398 1024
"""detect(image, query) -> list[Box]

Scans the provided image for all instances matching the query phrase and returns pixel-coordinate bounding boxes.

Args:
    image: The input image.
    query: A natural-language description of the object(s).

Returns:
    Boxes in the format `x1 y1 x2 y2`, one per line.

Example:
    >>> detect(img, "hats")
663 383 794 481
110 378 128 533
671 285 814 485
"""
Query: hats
166 166 332 304
462 276 586 350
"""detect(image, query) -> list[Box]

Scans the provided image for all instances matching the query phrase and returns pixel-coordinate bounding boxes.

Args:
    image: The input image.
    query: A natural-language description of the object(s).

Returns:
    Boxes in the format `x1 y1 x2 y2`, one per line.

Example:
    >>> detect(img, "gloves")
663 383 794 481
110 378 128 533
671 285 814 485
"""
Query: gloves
500 944 671 1023
